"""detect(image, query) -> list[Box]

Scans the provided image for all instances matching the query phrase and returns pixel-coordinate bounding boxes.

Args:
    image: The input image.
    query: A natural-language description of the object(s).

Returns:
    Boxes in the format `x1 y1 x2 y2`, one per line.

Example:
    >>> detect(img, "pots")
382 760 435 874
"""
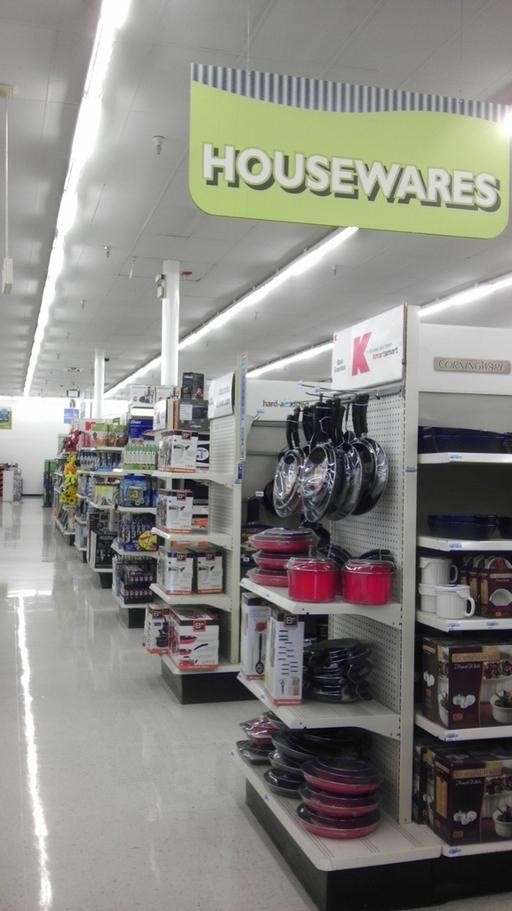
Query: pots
179 643 209 667
270 394 389 523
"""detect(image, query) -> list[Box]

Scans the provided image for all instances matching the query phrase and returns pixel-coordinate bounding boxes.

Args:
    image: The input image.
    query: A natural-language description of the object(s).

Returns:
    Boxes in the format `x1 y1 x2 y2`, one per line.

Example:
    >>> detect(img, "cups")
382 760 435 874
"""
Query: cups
418 557 475 620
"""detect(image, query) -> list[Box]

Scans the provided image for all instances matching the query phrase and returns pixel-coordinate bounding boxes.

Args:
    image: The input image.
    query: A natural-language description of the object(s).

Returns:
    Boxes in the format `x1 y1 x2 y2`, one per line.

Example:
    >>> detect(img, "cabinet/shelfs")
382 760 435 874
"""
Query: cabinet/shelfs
51 302 512 911
0 463 23 502
